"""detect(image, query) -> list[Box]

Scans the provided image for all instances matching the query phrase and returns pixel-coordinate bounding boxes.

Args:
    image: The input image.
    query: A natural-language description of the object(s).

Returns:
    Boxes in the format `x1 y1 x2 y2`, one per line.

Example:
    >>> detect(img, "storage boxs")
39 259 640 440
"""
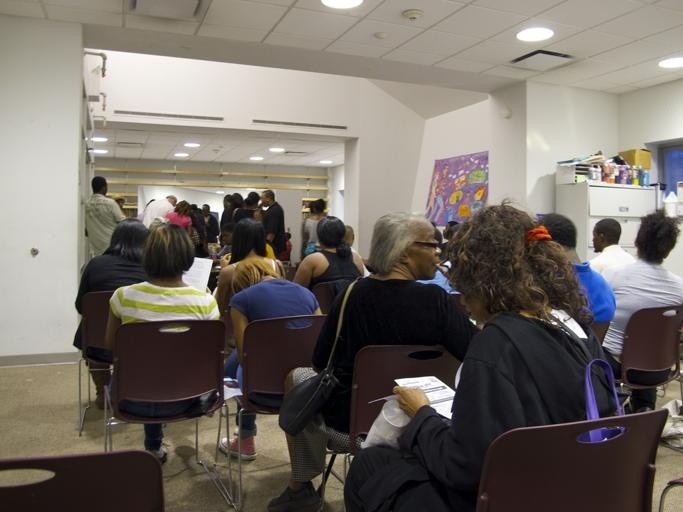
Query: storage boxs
617 148 651 170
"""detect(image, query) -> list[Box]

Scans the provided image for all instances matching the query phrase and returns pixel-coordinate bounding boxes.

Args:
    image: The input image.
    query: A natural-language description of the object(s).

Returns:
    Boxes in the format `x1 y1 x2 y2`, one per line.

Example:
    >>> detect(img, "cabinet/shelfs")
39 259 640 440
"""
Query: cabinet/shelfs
554 182 661 263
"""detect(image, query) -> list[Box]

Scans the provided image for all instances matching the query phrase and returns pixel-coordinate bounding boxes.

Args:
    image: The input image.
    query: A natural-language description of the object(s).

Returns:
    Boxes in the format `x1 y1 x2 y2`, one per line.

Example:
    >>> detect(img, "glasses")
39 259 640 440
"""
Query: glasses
412 240 440 251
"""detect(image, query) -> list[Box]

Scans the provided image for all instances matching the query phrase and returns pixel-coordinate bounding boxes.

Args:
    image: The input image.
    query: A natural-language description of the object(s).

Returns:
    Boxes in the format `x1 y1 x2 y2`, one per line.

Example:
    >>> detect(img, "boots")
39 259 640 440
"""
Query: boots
86 361 113 411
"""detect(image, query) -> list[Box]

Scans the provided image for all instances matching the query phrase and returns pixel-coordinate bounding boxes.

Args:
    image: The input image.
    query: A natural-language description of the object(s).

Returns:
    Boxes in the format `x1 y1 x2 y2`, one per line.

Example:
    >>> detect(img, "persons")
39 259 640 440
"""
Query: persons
539 209 682 417
75 177 364 461
343 197 620 512
268 211 481 512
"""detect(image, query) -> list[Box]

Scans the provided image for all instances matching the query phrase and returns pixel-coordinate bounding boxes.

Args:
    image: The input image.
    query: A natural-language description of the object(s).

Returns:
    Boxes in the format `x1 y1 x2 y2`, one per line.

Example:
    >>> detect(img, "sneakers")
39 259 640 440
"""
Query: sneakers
266 480 323 511
218 437 257 461
145 444 168 466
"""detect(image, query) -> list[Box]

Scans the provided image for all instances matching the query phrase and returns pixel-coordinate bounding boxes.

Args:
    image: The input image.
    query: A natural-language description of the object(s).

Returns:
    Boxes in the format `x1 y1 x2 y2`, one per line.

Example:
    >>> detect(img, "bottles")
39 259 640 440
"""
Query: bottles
590 164 652 187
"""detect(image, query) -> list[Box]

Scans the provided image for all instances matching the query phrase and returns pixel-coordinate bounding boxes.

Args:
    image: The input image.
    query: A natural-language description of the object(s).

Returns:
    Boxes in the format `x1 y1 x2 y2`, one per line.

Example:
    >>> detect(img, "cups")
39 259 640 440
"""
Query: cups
362 400 413 449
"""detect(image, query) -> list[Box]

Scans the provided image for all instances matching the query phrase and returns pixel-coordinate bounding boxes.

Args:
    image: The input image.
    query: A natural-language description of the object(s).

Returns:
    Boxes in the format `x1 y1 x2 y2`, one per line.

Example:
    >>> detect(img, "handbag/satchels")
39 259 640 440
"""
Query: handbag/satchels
278 276 364 439
575 359 627 443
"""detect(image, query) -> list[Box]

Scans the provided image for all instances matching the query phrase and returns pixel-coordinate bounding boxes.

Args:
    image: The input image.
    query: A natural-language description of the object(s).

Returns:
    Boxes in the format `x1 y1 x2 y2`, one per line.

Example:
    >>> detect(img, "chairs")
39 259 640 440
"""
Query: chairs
0 451 166 512
